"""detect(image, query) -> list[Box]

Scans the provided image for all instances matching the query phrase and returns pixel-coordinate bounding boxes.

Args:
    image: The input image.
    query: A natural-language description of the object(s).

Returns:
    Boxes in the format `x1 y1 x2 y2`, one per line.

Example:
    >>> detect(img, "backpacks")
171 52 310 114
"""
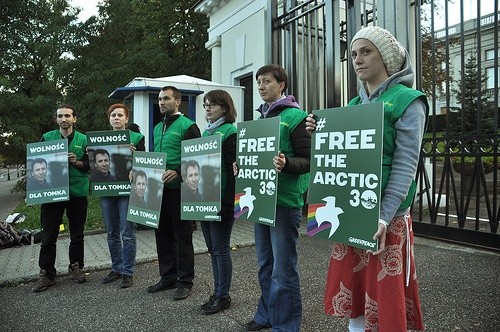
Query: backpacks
0 219 44 249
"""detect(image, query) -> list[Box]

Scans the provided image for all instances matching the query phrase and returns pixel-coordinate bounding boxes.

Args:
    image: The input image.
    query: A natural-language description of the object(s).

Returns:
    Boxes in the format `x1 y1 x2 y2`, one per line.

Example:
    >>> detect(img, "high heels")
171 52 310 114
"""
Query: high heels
203 295 231 314
200 297 217 310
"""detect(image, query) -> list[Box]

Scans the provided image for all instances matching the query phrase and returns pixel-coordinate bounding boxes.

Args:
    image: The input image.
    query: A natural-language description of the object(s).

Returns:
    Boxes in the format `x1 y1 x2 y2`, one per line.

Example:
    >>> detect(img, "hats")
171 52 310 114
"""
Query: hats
350 26 405 76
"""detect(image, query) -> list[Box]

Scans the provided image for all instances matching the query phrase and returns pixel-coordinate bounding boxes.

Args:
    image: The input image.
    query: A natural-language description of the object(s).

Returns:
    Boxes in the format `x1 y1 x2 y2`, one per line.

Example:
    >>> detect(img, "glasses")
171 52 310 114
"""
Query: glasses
203 103 222 109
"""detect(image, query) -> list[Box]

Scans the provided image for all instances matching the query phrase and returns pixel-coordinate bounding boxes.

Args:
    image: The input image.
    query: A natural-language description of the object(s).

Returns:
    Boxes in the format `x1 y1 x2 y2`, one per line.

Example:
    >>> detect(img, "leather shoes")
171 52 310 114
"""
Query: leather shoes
244 319 269 330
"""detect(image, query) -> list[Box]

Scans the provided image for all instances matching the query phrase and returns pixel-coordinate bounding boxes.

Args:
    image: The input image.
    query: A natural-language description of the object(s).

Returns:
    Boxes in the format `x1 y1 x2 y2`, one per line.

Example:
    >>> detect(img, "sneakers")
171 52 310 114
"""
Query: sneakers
68 261 86 283
103 271 122 284
147 281 170 293
174 287 191 300
121 274 133 288
32 269 56 292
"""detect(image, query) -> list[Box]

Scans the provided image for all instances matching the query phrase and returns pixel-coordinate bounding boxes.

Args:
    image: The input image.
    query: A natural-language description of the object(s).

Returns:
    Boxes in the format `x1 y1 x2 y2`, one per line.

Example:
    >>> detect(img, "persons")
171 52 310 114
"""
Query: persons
89 148 119 182
182 160 204 202
26 158 52 190
129 170 148 209
304 25 431 332
232 65 311 332
128 85 202 301
200 89 237 317
86 102 146 289
24 104 90 293
